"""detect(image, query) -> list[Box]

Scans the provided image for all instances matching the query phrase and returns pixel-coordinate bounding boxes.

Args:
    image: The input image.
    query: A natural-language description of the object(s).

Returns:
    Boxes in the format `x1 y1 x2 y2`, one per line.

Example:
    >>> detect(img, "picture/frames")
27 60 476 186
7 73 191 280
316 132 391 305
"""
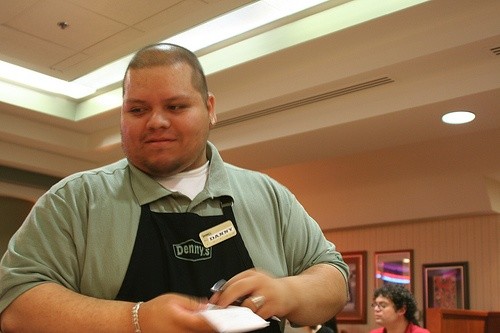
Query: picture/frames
373 250 414 296
336 251 368 325
422 261 470 329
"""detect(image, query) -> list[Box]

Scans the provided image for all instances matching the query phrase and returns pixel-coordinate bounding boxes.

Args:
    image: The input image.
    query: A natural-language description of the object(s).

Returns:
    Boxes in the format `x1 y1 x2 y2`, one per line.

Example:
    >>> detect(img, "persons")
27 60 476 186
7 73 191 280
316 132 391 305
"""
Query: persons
285 283 431 333
0 43 351 333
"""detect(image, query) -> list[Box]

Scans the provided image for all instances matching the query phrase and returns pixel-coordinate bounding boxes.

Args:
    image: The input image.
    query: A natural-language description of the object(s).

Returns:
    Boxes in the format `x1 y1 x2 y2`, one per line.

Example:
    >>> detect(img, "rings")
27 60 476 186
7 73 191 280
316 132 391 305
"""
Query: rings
249 293 265 308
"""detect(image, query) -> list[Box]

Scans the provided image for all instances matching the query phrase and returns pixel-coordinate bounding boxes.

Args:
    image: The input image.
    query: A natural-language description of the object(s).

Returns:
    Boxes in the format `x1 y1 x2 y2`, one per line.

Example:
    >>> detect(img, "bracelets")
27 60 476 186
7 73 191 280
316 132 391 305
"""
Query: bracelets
131 301 143 333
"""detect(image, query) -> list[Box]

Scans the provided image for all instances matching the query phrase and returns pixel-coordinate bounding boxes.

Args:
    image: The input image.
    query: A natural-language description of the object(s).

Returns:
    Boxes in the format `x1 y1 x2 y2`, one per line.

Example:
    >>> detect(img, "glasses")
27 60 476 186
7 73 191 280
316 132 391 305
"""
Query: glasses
371 301 399 309
